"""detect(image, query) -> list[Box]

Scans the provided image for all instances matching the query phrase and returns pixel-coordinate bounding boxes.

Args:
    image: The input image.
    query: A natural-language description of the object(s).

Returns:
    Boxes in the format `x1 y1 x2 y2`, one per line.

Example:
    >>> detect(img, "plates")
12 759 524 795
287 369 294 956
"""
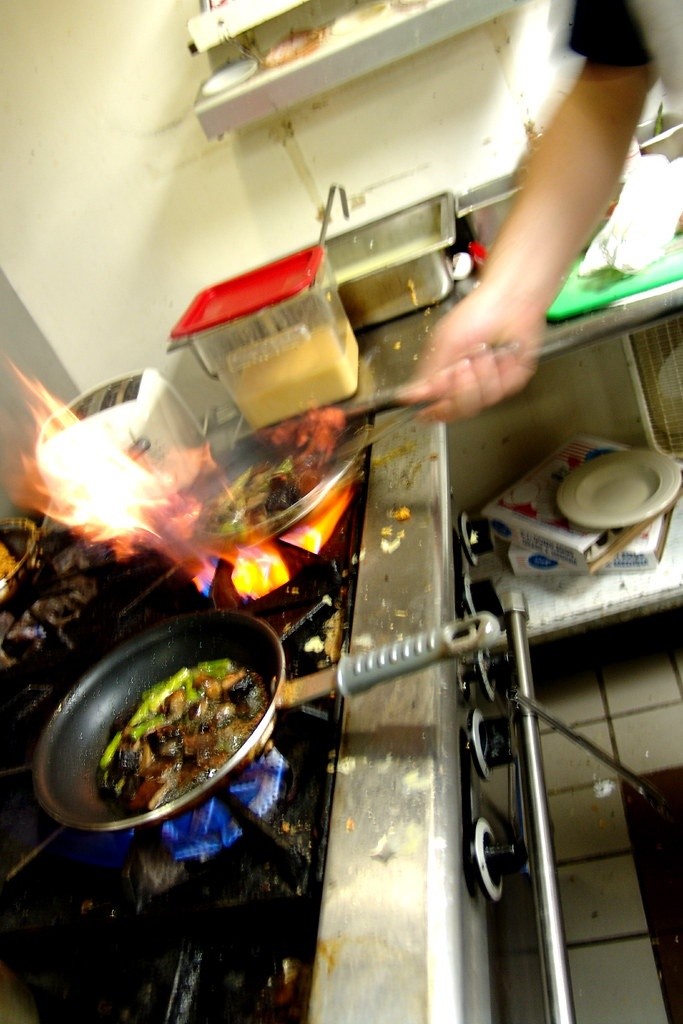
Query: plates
556 449 682 528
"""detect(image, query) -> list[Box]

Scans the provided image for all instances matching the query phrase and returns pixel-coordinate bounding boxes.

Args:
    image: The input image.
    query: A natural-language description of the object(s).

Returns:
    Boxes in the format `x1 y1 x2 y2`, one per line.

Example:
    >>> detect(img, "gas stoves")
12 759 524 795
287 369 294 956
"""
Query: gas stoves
0 398 547 1024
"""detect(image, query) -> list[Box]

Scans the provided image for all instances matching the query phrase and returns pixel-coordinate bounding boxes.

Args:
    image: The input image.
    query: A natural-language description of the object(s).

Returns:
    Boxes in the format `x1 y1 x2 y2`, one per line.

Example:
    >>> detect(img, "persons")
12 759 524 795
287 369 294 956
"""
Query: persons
395 0 682 429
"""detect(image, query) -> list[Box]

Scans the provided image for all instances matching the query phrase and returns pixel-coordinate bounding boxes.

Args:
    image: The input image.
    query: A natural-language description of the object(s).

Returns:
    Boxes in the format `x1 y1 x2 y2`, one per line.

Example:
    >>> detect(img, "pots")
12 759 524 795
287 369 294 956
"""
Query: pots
181 387 427 545
30 611 503 834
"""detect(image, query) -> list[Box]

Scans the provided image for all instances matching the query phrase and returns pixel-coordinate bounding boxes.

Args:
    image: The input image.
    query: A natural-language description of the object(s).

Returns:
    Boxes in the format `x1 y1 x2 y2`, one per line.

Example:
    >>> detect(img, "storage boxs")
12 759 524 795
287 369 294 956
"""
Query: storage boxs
168 244 360 428
508 507 673 575
482 433 683 574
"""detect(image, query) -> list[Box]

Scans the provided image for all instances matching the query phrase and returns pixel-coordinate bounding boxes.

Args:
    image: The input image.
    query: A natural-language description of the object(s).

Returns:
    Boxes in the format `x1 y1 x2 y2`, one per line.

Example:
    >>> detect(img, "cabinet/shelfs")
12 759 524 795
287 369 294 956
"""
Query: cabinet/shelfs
231 268 683 651
